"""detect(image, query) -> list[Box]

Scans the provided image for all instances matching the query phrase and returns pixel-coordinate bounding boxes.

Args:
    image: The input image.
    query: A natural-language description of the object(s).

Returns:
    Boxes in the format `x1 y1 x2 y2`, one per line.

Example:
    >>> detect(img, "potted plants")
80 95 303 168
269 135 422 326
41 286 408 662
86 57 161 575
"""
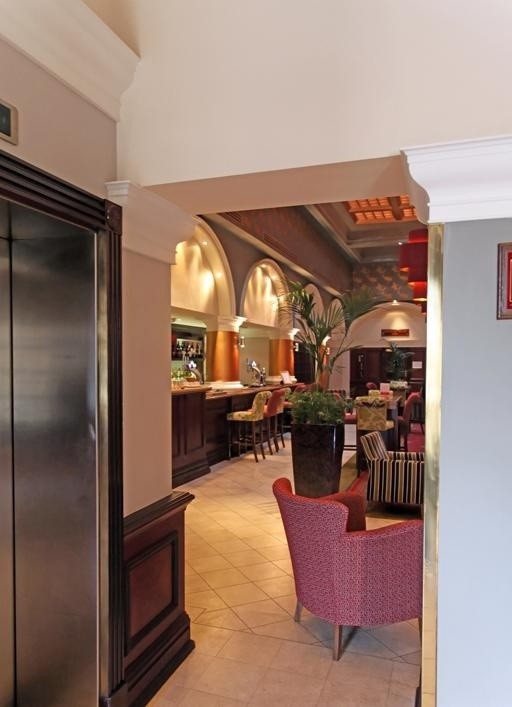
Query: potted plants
275 271 388 499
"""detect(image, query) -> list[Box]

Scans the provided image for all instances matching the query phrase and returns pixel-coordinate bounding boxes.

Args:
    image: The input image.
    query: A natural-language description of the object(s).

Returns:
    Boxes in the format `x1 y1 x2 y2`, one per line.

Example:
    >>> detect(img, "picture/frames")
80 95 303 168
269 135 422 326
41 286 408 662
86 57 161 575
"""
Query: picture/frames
497 242 512 319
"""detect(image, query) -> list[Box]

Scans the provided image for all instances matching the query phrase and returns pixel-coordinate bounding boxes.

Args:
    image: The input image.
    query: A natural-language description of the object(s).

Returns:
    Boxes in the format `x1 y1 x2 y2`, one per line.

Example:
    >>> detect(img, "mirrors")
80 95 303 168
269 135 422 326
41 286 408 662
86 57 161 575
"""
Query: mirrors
171 323 207 390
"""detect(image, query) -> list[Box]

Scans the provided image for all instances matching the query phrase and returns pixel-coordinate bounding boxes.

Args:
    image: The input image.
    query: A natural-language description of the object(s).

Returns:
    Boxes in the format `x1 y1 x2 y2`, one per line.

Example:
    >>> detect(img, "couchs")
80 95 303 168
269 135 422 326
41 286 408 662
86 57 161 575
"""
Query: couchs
357 430 424 514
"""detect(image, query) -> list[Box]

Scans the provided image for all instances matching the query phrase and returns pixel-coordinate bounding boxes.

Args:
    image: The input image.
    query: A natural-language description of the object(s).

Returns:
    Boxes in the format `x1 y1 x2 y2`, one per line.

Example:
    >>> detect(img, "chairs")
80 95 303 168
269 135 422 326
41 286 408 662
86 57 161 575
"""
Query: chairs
226 390 272 464
398 392 420 451
267 478 422 660
260 389 286 454
330 393 356 457
286 385 307 416
356 394 395 476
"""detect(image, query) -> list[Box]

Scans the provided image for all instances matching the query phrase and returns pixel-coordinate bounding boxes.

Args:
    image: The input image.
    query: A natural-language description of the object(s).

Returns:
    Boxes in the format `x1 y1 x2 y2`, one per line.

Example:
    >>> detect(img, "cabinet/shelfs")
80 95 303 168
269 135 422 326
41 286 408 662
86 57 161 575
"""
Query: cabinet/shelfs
172 392 211 488
